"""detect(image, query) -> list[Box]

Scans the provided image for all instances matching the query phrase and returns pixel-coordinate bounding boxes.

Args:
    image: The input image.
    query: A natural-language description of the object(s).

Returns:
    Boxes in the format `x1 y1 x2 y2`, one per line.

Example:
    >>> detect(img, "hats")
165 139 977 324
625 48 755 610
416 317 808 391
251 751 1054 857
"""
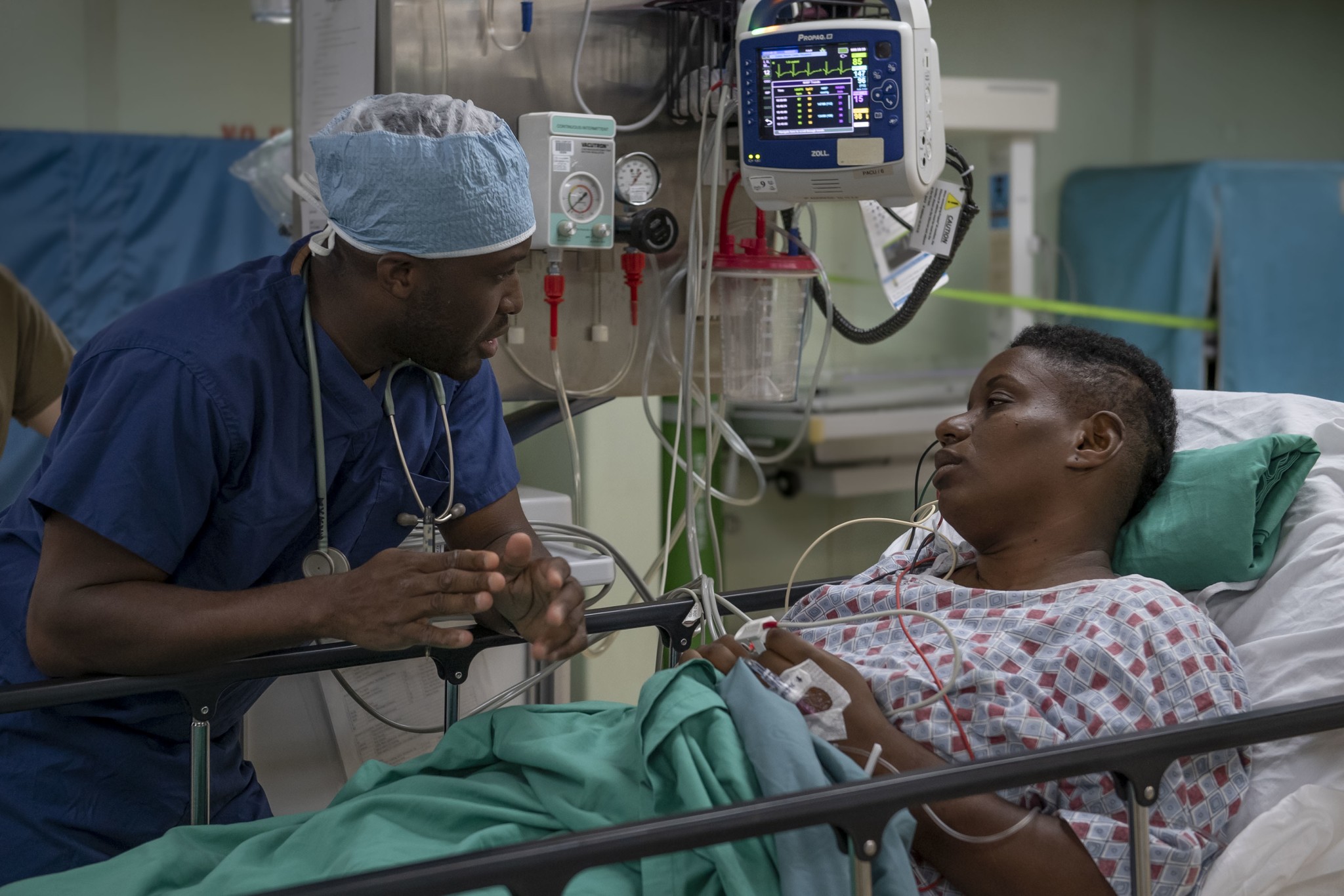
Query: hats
308 89 538 260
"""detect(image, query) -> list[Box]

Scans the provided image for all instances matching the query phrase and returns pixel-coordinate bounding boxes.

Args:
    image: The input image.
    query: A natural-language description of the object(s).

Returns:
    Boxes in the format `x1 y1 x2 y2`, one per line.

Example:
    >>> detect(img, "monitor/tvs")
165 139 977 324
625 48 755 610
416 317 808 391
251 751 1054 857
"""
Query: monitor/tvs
757 41 871 141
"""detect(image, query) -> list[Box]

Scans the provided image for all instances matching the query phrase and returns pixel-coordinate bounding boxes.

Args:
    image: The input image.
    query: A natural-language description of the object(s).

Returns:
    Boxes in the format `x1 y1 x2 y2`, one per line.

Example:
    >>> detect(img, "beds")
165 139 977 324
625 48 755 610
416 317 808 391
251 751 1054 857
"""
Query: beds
0 388 1344 896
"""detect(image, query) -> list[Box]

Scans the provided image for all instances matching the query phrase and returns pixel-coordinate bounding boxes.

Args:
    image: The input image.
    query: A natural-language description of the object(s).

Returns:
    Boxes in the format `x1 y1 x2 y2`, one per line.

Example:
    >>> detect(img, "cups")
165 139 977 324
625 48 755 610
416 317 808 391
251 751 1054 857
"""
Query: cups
712 251 818 402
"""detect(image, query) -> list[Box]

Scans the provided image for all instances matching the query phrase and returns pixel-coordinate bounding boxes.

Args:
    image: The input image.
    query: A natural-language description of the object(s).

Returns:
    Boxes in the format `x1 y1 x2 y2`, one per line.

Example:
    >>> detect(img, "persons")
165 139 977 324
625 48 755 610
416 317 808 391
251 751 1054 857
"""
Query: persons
1 92 592 883
0 263 79 451
0 324 1250 896
